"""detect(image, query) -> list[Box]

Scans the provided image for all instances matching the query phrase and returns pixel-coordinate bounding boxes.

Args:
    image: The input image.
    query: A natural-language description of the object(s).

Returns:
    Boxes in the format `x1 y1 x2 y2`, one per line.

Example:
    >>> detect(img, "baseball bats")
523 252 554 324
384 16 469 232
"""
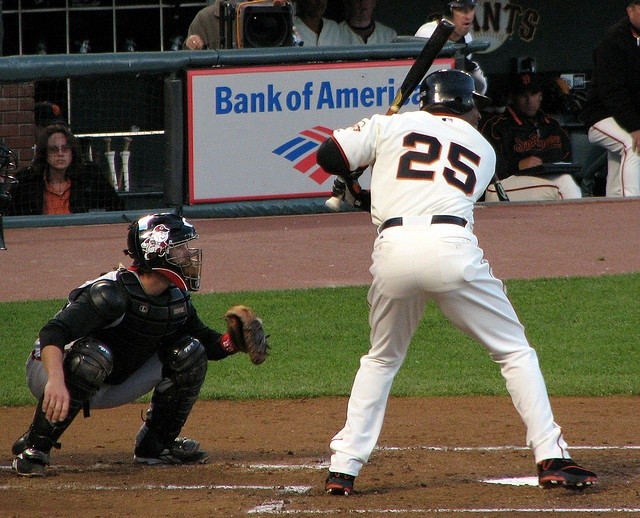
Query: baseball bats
325 19 456 212
117 137 132 192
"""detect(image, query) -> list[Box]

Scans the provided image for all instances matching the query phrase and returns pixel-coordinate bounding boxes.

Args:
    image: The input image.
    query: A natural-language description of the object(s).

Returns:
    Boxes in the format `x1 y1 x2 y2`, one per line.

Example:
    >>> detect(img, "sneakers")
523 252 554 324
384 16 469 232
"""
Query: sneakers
11 438 51 476
537 457 599 489
133 437 211 465
325 472 355 495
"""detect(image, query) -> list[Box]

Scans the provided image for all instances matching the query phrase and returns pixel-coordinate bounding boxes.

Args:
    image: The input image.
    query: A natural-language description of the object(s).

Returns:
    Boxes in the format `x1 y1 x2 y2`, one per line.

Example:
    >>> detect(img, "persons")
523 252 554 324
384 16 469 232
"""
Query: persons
182 1 236 50
12 213 272 477
338 0 398 46
0 120 128 216
585 0 640 198
413 0 487 96
316 69 600 496
477 71 582 201
292 0 341 47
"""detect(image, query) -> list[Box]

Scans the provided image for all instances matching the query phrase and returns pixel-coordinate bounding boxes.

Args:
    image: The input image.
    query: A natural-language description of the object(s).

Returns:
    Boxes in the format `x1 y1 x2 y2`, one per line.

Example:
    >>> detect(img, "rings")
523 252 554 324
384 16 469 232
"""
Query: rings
192 41 196 44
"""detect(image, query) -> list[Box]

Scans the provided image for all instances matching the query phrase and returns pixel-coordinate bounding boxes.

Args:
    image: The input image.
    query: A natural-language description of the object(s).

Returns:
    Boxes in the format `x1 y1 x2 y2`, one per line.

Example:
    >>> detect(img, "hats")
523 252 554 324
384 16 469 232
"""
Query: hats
514 71 540 96
35 101 67 125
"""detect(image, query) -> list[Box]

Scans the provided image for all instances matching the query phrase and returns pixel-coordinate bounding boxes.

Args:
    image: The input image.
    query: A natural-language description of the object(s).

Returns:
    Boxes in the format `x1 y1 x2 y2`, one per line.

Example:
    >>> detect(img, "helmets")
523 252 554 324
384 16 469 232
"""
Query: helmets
127 212 203 291
418 69 491 111
443 1 477 14
0 141 20 205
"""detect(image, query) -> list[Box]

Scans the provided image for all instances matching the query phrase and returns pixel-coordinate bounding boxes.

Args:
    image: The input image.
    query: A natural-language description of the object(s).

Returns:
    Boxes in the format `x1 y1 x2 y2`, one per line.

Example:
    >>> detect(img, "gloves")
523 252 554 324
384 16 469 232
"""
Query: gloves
472 62 487 95
332 172 364 209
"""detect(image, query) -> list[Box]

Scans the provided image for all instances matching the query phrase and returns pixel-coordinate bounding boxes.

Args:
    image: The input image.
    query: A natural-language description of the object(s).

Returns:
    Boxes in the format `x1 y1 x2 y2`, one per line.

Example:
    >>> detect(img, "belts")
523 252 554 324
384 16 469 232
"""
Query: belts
379 214 466 230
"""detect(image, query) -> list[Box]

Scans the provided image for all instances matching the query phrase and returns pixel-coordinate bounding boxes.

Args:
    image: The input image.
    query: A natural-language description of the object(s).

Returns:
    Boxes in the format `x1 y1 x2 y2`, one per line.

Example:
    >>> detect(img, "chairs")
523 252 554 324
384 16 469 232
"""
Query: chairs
570 134 608 197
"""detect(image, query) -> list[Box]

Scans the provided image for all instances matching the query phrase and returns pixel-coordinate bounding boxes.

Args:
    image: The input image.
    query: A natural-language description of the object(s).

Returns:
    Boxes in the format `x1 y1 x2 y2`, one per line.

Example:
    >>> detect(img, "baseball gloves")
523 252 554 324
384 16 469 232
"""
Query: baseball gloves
226 305 271 364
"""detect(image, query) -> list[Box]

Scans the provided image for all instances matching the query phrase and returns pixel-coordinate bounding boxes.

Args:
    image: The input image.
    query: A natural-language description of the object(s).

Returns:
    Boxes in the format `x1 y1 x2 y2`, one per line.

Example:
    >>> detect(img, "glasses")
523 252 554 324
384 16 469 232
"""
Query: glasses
44 144 70 154
